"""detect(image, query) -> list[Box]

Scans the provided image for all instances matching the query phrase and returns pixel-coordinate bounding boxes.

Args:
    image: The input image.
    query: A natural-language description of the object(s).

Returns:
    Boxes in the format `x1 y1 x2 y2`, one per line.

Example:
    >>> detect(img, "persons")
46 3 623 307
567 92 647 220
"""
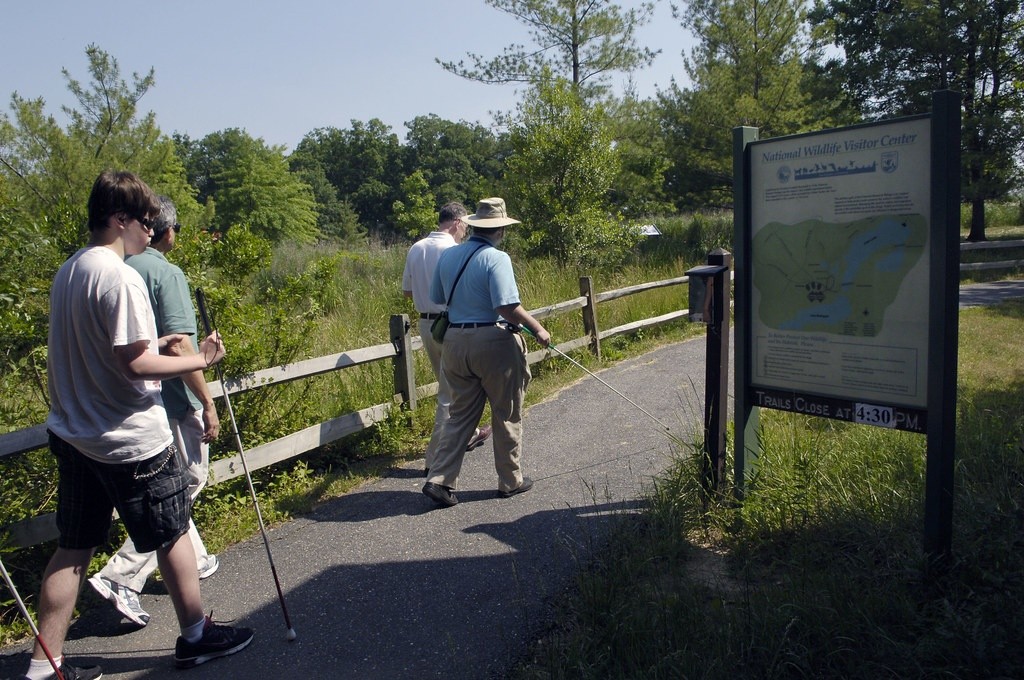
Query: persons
402 201 492 476
20 171 254 680
421 197 551 506
87 196 222 624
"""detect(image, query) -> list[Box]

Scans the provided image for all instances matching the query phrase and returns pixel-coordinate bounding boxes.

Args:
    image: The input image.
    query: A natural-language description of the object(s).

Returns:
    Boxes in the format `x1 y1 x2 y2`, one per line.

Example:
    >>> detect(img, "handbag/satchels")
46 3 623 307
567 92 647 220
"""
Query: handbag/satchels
429 311 448 344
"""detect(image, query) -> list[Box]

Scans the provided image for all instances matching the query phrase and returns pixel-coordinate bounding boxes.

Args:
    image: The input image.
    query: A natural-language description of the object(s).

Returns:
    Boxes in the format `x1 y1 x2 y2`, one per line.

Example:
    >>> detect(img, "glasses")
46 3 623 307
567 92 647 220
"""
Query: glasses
168 222 181 234
136 215 156 230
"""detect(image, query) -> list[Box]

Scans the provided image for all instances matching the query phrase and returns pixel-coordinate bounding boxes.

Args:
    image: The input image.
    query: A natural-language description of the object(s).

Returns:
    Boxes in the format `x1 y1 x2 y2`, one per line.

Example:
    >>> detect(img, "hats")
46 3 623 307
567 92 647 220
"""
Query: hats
462 196 522 228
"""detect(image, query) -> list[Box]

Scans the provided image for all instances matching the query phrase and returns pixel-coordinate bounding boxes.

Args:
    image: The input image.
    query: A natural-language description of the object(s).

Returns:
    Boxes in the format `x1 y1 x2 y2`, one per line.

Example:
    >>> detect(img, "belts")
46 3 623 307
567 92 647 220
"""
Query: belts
420 312 439 320
448 320 508 328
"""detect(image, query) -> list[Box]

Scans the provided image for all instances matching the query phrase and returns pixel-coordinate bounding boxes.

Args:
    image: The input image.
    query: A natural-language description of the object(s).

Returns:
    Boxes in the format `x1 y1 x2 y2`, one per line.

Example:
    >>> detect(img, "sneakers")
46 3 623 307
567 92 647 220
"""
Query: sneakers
88 572 150 625
196 552 220 579
173 608 253 668
19 653 103 680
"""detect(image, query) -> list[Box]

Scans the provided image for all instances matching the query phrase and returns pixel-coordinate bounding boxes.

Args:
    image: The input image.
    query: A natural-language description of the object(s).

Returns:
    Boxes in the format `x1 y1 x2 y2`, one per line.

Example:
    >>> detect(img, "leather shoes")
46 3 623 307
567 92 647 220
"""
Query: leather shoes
422 482 459 504
498 477 533 496
423 467 429 477
467 423 492 450
